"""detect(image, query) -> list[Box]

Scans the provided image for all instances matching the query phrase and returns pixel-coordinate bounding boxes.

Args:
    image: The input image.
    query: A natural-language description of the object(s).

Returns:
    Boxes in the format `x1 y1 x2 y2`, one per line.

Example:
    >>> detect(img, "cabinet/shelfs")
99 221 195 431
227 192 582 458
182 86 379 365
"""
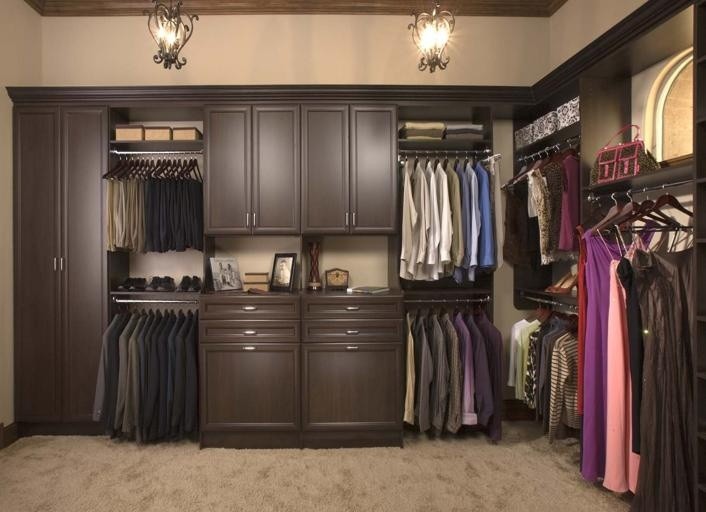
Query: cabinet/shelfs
301 284 404 446
198 289 303 448
9 102 106 439
299 103 398 237
204 102 302 235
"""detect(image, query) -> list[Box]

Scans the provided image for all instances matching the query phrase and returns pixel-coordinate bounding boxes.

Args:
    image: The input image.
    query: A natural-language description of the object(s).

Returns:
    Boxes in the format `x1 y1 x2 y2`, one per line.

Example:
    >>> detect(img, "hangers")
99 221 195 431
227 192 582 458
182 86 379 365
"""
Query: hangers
501 110 706 505
405 295 495 329
101 148 203 188
399 150 491 179
113 300 197 333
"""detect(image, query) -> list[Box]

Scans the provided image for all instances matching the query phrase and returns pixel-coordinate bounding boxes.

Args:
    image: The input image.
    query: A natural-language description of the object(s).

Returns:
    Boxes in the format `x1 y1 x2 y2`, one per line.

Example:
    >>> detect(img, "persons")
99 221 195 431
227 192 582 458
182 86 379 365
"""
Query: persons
278 260 290 286
216 262 233 287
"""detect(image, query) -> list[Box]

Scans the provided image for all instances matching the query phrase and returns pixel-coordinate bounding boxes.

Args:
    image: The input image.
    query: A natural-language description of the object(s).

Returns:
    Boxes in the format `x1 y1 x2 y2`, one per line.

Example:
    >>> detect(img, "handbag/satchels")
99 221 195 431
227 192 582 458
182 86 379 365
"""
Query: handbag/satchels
588 124 661 184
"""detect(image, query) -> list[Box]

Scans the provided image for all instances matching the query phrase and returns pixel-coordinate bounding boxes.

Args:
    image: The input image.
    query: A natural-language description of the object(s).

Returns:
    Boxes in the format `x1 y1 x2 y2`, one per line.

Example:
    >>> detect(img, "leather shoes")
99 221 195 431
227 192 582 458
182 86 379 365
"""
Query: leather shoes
544 271 578 293
144 276 174 292
175 276 201 291
118 277 145 291
